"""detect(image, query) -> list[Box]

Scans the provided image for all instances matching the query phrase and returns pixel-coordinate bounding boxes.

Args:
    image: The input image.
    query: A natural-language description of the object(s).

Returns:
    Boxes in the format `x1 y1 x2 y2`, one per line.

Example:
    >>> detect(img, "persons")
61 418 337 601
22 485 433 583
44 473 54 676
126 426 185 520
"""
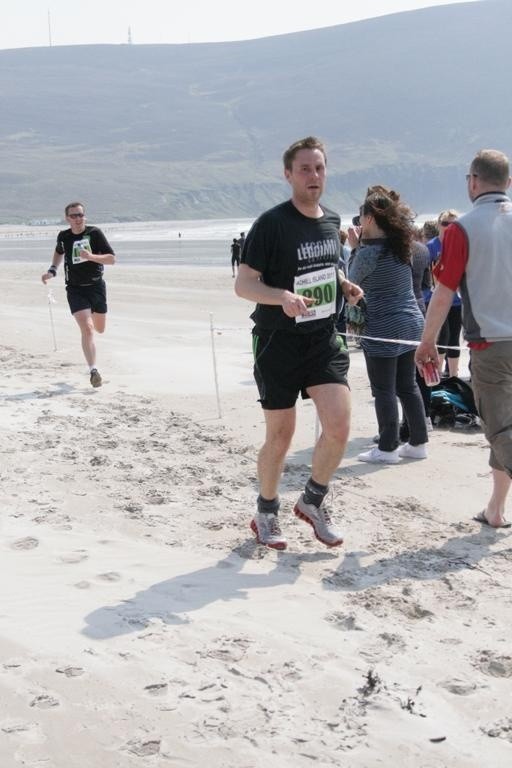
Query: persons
338 190 432 466
238 232 247 258
411 146 512 530
362 183 414 227
41 202 116 391
234 134 366 552
229 238 241 279
330 209 469 379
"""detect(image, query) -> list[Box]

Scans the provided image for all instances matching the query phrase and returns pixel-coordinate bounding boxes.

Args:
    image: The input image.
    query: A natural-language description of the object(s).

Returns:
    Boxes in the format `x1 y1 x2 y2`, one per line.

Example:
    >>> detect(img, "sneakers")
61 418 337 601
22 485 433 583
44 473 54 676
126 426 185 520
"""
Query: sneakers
294 493 344 547
90 370 102 387
358 432 428 465
251 512 287 549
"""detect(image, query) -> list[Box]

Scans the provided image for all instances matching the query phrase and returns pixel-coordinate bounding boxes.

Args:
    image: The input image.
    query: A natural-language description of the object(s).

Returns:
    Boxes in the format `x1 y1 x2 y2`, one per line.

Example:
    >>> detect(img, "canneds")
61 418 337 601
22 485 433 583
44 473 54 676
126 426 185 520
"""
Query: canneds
421 358 442 387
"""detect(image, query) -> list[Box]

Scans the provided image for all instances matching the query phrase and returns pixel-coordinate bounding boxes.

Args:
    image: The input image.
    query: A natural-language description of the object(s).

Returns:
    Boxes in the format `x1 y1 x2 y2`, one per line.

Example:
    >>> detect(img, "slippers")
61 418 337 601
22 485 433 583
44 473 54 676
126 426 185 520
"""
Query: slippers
473 509 512 527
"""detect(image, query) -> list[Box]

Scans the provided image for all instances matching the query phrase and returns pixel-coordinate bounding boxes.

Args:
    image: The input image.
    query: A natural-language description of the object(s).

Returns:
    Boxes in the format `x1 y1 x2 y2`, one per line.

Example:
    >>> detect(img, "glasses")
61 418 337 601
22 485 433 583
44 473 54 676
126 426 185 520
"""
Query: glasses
465 173 478 180
68 213 84 219
441 221 449 227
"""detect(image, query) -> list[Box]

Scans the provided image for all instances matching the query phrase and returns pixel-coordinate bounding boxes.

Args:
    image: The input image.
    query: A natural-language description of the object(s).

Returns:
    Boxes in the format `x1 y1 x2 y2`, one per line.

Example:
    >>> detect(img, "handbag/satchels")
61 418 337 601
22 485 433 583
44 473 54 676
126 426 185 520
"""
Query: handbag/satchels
344 295 366 326
427 376 480 431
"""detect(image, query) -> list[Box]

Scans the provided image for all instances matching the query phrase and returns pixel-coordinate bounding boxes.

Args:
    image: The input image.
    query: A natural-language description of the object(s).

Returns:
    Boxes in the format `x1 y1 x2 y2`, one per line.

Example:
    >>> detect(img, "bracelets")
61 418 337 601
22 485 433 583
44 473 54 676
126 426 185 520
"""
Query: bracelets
48 265 58 277
